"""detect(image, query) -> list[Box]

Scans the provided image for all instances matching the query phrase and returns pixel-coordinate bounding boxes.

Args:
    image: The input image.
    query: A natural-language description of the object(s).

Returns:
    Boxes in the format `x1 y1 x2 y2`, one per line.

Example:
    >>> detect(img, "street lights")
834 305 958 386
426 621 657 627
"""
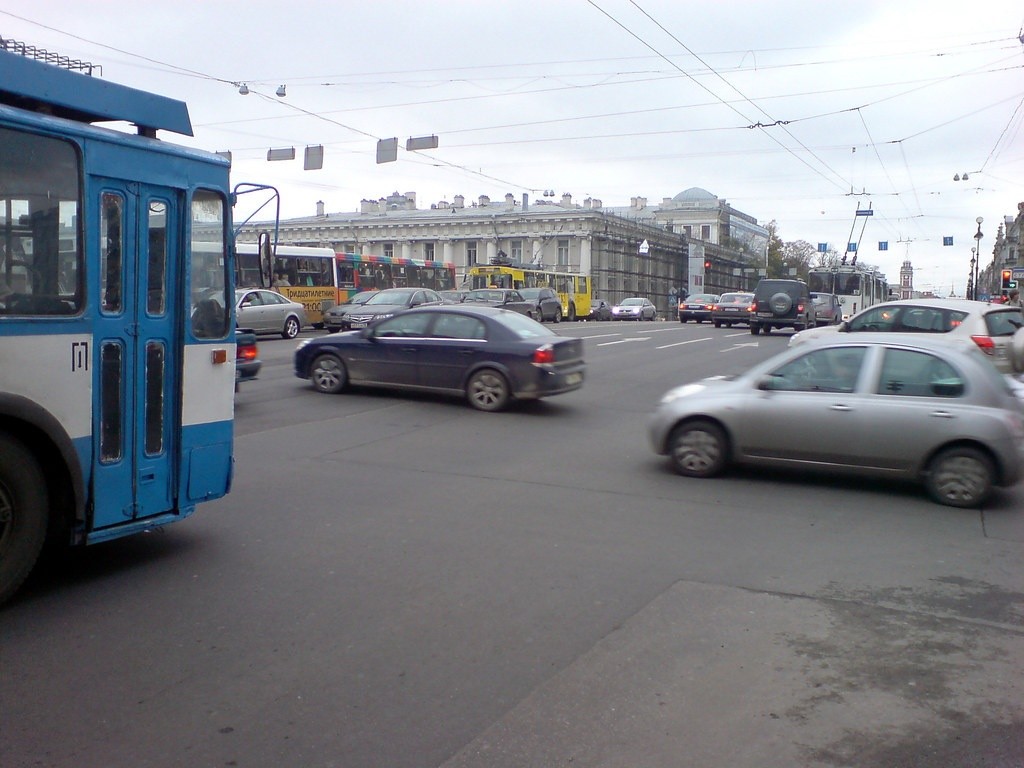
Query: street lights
973 216 983 301
968 245 977 300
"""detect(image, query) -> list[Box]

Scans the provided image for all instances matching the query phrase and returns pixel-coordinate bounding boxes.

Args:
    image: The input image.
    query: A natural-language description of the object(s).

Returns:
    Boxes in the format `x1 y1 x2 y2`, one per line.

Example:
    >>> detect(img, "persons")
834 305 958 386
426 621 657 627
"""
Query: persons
837 354 859 391
1004 290 1024 307
242 258 330 286
248 294 259 305
339 264 452 289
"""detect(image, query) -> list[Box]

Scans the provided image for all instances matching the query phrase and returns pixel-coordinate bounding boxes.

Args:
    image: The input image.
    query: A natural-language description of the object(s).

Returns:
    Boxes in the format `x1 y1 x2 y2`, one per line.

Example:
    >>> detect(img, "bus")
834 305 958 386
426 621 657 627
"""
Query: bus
467 249 592 323
807 201 890 323
0 34 283 612
97 233 458 332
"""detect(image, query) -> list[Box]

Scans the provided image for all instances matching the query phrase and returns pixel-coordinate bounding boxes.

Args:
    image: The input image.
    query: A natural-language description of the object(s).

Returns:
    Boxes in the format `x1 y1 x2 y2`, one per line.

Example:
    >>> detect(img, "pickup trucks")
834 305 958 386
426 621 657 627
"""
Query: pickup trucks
234 326 263 394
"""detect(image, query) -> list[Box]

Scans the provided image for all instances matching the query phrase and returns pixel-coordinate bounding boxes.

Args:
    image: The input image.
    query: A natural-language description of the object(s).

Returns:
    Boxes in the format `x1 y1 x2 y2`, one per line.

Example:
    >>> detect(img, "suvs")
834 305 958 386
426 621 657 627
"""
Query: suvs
748 275 819 336
787 297 1024 386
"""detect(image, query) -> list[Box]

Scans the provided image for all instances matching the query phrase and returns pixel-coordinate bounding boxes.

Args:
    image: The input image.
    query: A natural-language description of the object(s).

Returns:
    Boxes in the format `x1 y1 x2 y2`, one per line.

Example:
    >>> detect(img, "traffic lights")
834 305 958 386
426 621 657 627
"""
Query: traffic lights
705 261 710 268
1001 269 1019 290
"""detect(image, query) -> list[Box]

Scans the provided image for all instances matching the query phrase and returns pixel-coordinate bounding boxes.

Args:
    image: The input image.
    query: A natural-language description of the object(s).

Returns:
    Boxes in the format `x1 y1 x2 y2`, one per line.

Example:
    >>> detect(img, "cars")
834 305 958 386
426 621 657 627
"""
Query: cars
681 294 722 325
590 298 613 321
712 292 756 328
190 287 307 339
612 297 658 322
290 303 587 413
322 282 564 335
647 330 1024 507
809 291 842 326
